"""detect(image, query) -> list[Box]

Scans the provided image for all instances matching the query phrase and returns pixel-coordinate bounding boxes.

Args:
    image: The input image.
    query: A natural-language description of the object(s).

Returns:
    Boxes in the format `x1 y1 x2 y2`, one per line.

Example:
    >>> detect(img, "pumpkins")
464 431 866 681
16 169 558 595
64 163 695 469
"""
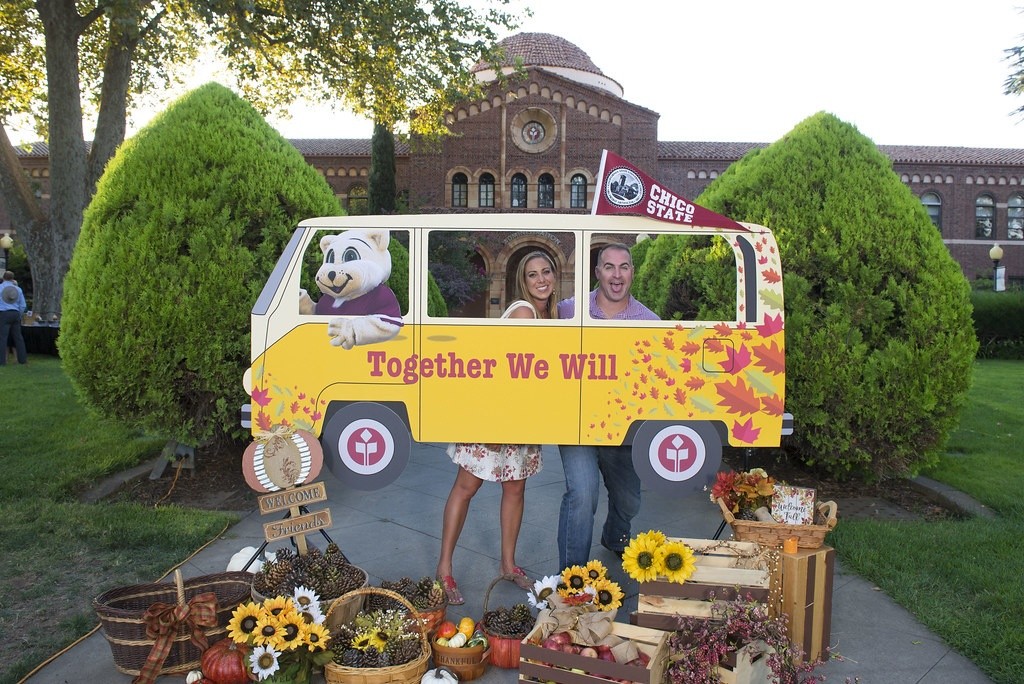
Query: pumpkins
421 666 458 684
226 546 278 574
186 638 251 684
436 617 487 648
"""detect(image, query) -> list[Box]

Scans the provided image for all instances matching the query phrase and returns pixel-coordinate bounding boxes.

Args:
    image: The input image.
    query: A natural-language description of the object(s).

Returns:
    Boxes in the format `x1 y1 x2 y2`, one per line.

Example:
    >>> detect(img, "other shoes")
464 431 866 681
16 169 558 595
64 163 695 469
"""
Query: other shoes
601 535 626 560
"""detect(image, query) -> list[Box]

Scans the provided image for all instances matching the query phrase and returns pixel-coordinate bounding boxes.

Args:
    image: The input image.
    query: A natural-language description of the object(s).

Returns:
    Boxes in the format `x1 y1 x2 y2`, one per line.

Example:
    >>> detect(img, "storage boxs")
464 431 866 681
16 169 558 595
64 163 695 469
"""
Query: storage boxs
519 622 668 684
631 537 835 684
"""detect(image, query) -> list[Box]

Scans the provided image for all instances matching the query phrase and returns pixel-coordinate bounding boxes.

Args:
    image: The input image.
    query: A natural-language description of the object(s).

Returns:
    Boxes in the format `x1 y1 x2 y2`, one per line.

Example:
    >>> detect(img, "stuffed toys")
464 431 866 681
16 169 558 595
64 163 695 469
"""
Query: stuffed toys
299 230 405 349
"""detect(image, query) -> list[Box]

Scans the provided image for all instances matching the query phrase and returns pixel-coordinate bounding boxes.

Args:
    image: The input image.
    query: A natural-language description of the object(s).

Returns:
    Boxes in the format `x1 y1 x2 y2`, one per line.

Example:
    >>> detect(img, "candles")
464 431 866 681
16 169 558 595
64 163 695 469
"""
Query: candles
784 537 798 553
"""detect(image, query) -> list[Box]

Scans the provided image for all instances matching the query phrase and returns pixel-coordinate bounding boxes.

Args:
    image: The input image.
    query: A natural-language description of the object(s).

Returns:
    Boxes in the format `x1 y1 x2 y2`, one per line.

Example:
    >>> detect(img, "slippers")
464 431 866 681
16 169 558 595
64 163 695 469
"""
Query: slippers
498 566 534 590
437 577 466 605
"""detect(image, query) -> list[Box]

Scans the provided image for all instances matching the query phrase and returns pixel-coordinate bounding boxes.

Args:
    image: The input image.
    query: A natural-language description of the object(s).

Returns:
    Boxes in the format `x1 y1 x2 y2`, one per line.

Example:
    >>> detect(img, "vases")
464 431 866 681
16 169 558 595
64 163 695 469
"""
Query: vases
754 507 778 523
253 660 310 684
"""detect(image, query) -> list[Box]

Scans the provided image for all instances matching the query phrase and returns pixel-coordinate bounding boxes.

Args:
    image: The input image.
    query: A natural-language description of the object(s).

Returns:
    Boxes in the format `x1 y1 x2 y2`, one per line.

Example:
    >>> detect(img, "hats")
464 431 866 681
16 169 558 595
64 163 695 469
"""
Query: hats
2 286 18 304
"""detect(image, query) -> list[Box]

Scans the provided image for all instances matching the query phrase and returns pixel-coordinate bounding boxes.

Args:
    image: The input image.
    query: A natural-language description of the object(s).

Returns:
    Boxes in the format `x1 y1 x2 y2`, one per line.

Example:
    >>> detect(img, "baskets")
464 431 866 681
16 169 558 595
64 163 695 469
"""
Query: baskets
92 569 254 676
322 588 431 684
716 499 837 550
404 590 448 641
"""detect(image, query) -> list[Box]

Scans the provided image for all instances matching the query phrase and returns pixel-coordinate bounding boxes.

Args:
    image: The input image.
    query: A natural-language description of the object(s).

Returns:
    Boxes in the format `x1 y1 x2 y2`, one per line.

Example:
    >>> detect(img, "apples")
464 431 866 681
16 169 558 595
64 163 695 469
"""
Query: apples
540 632 650 684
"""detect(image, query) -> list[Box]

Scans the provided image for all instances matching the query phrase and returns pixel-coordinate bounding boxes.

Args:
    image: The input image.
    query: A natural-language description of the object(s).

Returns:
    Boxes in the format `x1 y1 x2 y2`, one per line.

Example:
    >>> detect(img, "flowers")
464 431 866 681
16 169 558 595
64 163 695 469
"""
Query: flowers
703 468 780 520
252 543 366 600
525 559 626 615
330 607 431 668
660 584 860 684
225 585 333 684
485 602 532 636
368 575 444 608
622 528 783 610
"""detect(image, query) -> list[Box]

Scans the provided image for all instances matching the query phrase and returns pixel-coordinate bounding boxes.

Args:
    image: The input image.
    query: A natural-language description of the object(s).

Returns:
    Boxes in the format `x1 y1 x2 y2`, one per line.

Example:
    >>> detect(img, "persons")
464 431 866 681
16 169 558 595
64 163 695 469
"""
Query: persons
437 251 558 605
0 271 27 365
556 243 661 572
